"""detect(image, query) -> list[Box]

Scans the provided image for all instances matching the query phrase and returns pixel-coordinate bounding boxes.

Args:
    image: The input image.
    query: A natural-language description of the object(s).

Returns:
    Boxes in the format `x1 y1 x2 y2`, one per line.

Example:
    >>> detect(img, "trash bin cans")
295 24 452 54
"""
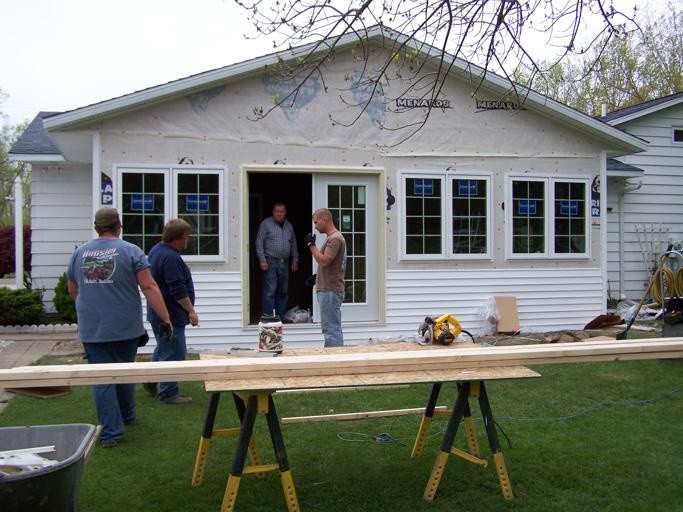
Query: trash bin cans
0 423 105 512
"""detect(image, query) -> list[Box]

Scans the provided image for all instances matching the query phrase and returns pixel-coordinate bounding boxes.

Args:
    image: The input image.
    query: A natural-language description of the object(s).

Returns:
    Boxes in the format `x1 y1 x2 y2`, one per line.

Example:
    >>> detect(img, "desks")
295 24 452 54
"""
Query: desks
191 341 542 512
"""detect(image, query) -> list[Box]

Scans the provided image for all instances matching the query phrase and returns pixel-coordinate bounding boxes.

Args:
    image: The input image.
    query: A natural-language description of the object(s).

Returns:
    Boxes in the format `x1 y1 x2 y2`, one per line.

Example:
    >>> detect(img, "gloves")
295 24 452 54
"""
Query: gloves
156 321 174 340
305 233 316 247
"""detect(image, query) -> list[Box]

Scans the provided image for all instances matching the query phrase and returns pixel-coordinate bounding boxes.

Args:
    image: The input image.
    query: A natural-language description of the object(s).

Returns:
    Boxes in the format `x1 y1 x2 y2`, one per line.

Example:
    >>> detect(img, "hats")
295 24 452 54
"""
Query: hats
95 207 119 227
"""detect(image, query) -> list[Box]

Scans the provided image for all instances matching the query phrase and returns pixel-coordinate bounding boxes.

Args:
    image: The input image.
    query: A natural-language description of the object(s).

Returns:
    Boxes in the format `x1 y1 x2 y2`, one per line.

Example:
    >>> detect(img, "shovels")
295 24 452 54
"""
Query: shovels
615 243 673 340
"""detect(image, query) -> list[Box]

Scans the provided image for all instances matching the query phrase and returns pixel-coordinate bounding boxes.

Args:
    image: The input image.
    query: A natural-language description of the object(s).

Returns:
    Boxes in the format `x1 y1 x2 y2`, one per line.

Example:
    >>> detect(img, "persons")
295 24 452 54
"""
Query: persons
304 207 347 348
139 218 200 404
254 199 299 324
64 206 174 448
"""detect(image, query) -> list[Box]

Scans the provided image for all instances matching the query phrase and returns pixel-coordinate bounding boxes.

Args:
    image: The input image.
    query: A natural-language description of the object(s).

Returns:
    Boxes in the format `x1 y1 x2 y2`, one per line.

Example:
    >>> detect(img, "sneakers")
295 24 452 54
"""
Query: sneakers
166 395 192 404
143 383 158 395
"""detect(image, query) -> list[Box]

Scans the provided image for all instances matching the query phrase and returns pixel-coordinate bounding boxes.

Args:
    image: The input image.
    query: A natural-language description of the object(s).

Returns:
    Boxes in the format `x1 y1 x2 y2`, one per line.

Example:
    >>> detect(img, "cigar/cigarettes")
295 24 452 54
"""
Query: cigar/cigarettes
196 324 199 327
